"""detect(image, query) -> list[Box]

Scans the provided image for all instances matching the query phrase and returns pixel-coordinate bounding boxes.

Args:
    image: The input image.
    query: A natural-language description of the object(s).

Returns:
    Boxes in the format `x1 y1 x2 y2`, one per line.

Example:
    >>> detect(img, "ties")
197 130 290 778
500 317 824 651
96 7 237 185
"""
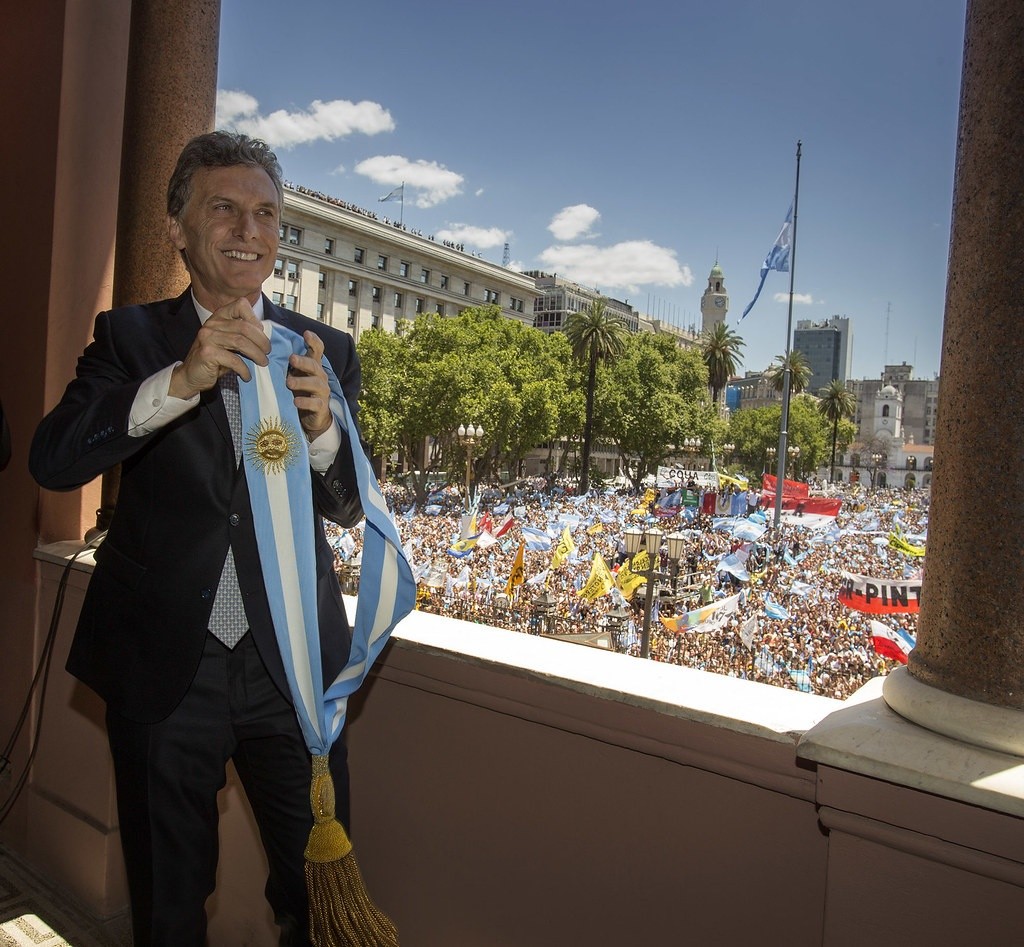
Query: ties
207 367 251 651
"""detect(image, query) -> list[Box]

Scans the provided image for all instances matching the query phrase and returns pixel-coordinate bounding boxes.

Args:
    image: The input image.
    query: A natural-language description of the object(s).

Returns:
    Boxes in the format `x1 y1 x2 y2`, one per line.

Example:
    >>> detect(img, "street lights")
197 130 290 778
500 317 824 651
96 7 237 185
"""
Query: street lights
906 455 914 493
682 437 702 484
870 453 882 492
458 422 484 512
622 525 686 657
788 446 801 485
766 447 776 474
724 442 736 475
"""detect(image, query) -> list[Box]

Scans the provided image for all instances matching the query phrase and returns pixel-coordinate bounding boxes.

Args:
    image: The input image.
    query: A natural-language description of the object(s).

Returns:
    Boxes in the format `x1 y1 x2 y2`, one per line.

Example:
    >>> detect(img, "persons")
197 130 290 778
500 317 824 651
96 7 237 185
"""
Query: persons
321 476 931 702
28 130 360 947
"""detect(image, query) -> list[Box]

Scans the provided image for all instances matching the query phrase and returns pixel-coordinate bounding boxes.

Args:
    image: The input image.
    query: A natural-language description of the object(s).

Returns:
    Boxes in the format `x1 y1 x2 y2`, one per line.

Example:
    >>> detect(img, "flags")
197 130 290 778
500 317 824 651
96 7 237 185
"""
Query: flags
445 532 484 559
615 546 661 601
737 195 793 323
868 618 916 665
576 552 619 602
551 524 574 568
501 538 525 599
492 511 513 536
